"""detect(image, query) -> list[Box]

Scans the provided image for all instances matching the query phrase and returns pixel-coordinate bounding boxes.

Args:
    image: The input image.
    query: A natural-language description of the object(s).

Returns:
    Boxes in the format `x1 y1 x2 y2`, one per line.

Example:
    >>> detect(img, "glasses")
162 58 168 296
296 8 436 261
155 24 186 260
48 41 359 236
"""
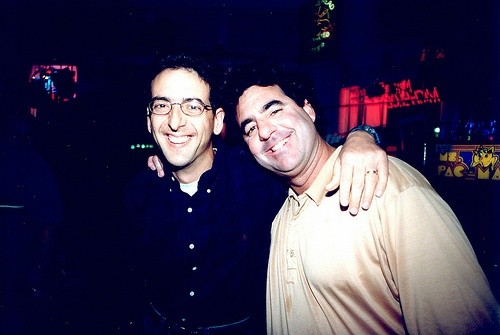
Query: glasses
148 99 212 115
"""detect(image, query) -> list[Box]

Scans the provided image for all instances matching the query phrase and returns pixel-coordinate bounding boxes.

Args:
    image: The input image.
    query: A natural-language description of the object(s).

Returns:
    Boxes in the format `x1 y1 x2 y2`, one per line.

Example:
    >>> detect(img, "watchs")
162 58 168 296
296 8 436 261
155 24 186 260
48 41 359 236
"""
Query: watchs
348 124 381 144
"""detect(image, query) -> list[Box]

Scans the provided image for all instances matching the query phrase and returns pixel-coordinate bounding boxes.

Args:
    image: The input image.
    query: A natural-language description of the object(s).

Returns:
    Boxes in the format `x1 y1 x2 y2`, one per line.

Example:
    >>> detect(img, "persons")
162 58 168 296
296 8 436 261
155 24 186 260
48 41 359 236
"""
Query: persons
115 53 500 335
